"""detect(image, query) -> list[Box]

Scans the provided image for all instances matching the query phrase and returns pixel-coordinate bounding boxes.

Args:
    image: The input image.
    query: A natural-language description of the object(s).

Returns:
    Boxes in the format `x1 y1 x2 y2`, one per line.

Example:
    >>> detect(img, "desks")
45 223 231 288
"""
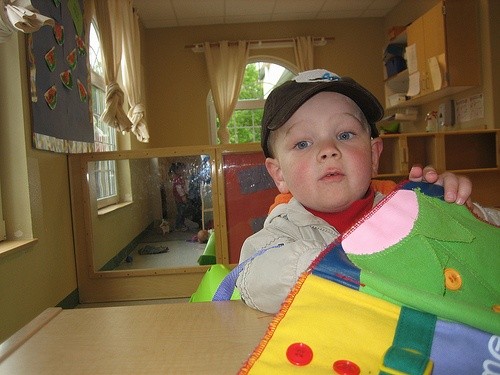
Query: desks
1 300 295 375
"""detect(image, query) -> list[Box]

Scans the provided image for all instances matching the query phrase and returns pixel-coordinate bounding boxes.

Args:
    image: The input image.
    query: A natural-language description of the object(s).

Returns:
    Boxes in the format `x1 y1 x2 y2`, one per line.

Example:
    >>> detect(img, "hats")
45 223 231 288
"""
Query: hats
260 68 384 147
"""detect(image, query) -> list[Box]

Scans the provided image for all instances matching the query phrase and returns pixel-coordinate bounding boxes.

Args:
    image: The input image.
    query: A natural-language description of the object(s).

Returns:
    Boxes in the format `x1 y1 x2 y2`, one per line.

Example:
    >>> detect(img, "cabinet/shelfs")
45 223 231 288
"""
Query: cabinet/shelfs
381 0 479 110
367 130 500 211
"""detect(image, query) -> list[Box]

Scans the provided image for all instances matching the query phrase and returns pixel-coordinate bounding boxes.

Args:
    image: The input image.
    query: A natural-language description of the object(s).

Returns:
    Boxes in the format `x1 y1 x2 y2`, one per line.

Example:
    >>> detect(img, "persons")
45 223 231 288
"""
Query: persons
237 65 473 319
168 162 190 232
